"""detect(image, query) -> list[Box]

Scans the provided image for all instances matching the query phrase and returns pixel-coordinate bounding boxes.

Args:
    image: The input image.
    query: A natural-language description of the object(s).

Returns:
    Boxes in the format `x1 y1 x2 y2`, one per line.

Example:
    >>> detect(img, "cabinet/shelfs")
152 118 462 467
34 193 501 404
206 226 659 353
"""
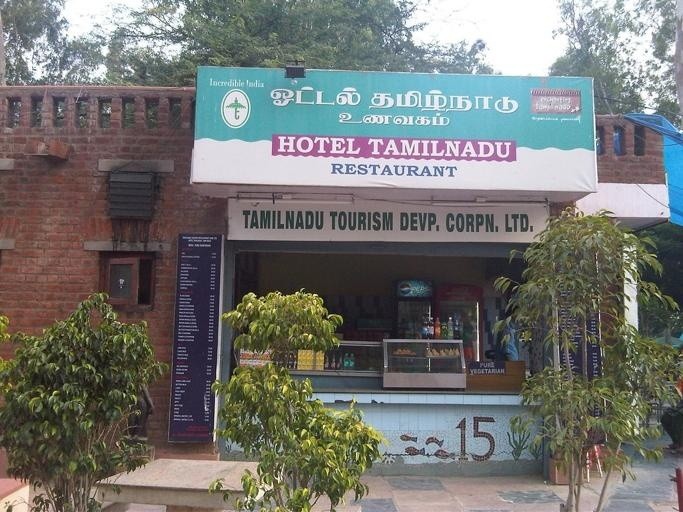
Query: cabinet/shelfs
285 338 467 390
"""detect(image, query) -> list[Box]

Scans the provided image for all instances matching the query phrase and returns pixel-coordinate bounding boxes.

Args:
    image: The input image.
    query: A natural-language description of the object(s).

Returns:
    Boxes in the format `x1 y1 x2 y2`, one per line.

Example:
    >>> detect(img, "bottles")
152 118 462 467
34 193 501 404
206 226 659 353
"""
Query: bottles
423 315 460 339
343 352 355 369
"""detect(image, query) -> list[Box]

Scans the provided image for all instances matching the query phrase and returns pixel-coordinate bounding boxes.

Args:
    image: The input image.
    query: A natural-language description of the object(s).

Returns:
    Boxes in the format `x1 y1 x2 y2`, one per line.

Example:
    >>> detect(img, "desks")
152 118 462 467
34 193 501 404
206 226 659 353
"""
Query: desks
95 458 285 512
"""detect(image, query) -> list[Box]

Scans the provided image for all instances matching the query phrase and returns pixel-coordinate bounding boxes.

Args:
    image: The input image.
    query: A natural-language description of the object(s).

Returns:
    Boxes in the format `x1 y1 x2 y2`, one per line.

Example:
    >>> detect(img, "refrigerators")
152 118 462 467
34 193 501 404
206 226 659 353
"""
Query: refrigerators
434 282 485 361
392 278 432 340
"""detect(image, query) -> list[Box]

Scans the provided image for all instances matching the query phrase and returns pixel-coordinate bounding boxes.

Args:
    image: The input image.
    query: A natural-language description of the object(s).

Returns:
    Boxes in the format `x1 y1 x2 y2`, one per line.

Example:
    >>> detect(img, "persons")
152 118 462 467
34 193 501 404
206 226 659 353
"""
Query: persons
660 399 683 455
495 303 521 361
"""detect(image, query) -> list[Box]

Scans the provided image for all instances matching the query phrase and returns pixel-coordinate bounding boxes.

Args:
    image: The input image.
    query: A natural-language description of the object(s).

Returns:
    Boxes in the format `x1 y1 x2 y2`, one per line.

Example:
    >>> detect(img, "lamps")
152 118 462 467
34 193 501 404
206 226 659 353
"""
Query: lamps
284 59 308 79
